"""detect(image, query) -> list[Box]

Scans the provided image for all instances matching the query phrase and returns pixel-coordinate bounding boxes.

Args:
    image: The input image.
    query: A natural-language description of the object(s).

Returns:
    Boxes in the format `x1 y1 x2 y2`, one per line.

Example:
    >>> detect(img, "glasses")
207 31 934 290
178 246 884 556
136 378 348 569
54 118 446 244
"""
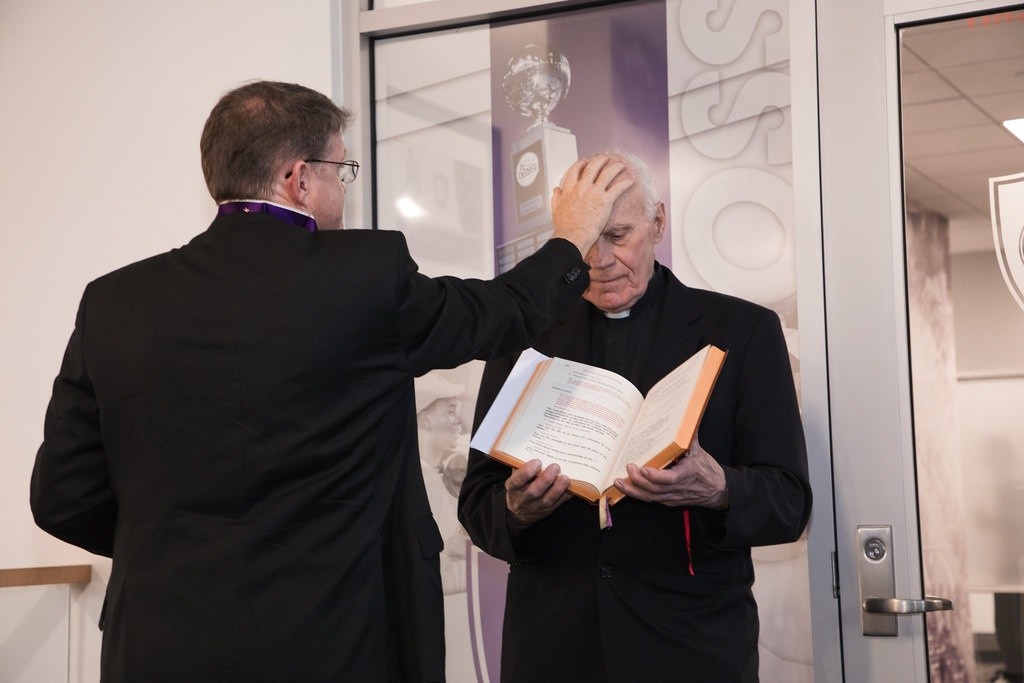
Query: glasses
285 158 360 183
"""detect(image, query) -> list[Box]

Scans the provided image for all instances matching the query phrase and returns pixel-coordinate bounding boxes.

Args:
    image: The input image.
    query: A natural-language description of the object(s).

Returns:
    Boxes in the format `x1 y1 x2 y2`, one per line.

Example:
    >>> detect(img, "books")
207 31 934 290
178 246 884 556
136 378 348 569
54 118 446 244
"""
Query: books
468 343 729 529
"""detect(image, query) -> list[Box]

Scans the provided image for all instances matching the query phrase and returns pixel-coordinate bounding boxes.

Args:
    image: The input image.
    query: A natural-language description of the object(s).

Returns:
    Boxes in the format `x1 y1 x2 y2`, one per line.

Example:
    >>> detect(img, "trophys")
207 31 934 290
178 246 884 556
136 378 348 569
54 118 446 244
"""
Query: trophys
498 44 578 273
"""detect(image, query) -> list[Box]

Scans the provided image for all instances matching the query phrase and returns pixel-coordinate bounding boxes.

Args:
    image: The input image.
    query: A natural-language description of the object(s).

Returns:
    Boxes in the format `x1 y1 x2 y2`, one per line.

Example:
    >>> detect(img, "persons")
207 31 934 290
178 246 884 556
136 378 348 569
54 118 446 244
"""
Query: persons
415 373 478 683
30 82 633 683
457 152 813 683
